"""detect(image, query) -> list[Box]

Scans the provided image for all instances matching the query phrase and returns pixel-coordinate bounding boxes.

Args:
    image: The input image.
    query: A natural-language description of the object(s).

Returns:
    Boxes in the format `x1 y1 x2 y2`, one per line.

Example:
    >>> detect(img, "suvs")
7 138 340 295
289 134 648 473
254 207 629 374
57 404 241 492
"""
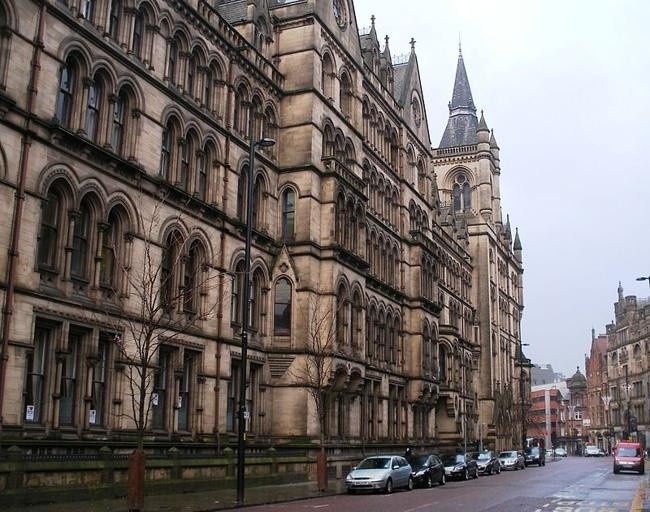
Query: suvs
612 441 646 474
584 445 599 456
523 446 546 466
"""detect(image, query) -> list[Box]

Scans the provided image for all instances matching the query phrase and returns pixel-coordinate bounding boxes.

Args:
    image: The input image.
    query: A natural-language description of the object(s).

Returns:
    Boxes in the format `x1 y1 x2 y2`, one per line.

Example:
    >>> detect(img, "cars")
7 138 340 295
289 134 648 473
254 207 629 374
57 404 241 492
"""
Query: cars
441 453 479 480
546 449 554 457
497 450 525 471
401 453 446 487
575 446 585 456
598 448 608 456
554 448 568 457
470 448 501 475
345 455 414 494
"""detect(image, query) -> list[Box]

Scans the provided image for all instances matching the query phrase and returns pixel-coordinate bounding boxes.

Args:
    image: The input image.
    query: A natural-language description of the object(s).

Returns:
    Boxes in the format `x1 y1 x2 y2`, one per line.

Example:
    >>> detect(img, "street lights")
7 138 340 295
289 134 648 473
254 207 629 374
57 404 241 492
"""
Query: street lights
236 136 276 504
603 382 608 434
520 342 529 451
623 364 630 439
636 276 650 289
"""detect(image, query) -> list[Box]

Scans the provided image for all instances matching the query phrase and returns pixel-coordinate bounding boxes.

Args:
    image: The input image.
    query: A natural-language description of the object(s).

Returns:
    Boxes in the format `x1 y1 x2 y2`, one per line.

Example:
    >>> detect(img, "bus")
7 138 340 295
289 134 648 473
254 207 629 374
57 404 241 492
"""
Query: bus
525 438 539 447
